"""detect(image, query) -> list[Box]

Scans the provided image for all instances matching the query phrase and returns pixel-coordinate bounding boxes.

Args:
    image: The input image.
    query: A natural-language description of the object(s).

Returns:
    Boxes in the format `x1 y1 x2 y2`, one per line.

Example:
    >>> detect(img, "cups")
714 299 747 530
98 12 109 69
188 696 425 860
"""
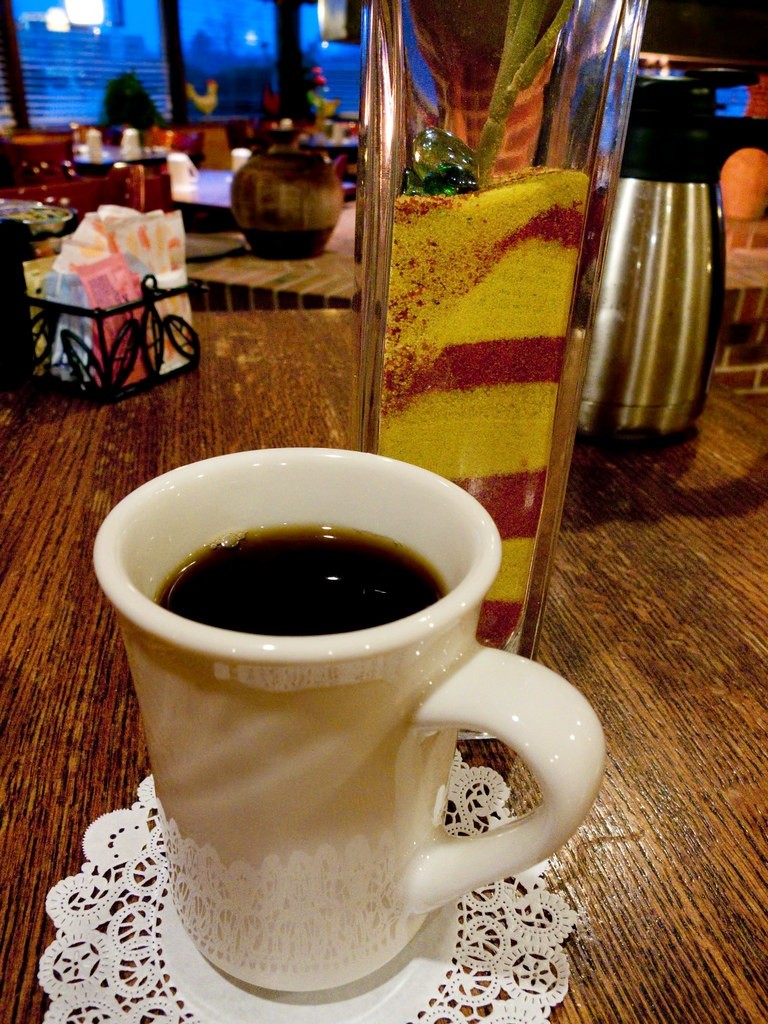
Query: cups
93 450 605 992
168 153 199 184
232 148 251 171
87 131 101 158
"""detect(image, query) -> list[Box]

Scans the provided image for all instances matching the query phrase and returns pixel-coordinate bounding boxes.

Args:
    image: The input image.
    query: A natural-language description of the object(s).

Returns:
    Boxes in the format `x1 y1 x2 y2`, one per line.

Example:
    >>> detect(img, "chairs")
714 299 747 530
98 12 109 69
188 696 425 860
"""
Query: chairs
1 160 145 223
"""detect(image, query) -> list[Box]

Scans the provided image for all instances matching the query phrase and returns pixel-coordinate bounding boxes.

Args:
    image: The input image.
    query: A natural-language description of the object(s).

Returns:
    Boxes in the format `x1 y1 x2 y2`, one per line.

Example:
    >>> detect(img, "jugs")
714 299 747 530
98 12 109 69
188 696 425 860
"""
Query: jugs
570 72 725 446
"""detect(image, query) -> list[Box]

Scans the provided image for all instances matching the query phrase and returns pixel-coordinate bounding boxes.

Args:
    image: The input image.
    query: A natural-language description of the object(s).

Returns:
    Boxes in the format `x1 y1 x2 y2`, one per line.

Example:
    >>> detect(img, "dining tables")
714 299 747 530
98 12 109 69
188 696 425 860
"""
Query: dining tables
74 144 170 176
0 308 768 1024
300 135 357 181
